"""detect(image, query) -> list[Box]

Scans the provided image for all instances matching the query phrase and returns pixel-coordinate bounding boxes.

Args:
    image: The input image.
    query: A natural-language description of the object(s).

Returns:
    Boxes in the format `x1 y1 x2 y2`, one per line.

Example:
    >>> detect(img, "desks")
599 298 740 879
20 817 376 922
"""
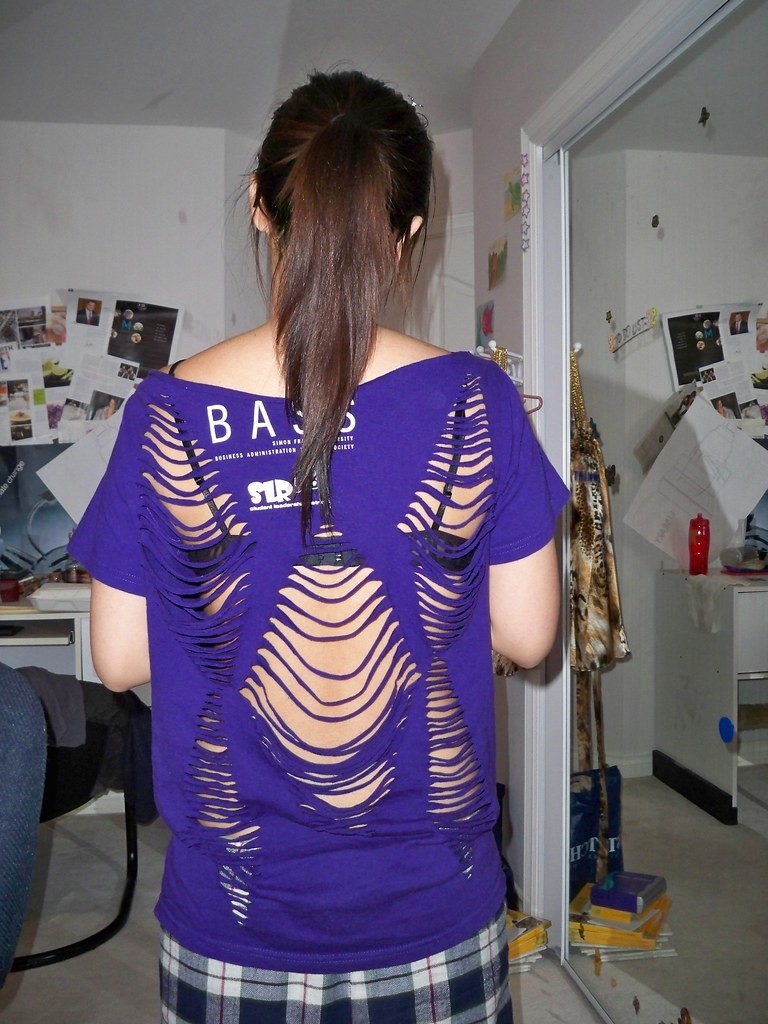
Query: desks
0 583 152 815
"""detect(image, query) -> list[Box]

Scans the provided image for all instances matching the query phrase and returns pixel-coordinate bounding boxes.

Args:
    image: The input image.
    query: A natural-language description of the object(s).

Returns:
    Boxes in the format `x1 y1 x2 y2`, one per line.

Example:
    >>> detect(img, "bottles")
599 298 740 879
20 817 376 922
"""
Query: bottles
689 513 710 576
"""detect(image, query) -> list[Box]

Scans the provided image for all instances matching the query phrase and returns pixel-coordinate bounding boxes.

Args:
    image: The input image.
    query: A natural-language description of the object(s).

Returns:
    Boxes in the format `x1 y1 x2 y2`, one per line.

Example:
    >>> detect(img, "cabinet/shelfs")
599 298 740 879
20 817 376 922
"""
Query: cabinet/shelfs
653 568 768 825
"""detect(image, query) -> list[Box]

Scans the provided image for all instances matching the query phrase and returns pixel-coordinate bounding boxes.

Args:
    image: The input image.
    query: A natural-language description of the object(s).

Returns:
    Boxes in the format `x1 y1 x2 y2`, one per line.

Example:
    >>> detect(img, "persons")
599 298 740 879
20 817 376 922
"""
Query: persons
77 297 100 326
729 312 748 335
93 397 120 420
68 69 571 1024
714 397 736 419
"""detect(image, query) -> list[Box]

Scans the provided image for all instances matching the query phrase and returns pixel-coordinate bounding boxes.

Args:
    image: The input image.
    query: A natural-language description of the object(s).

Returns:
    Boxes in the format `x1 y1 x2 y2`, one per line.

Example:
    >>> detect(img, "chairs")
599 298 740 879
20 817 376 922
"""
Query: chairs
0 770 141 974
